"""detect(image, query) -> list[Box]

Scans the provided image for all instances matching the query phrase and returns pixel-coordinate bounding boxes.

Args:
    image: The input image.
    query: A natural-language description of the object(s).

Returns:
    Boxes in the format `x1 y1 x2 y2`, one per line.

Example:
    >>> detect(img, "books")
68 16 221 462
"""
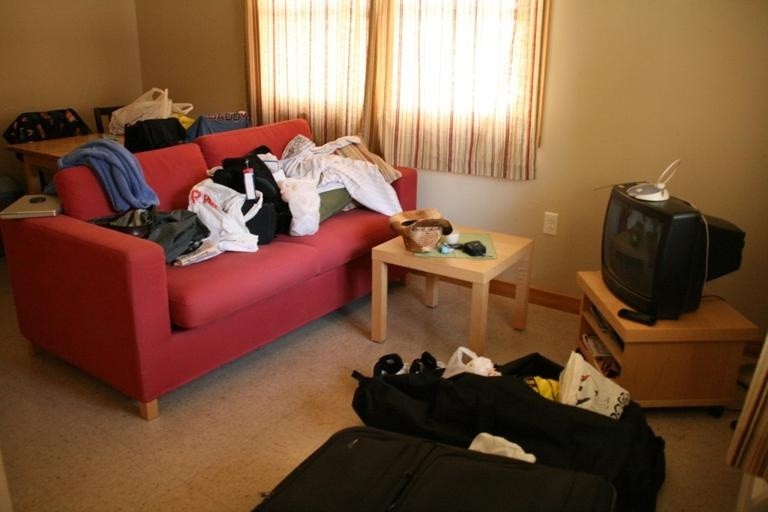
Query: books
1 193 62 219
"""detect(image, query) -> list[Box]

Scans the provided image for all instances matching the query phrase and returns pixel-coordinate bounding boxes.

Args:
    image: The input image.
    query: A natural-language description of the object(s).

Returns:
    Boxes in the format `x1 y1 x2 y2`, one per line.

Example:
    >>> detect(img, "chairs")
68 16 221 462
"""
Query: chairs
4 104 248 194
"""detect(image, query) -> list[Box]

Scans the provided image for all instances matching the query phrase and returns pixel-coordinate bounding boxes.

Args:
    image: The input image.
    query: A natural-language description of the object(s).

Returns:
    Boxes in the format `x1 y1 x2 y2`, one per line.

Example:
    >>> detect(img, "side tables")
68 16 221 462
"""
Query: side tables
369 221 535 366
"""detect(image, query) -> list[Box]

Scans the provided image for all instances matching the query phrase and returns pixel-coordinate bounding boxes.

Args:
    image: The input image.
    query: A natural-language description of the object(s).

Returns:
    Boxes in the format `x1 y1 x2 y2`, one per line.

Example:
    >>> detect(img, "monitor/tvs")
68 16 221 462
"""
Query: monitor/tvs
601 182 747 318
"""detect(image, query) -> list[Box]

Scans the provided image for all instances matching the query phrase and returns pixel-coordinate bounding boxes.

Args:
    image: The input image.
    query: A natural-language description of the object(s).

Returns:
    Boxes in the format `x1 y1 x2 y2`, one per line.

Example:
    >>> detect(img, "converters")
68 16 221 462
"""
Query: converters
463 240 486 256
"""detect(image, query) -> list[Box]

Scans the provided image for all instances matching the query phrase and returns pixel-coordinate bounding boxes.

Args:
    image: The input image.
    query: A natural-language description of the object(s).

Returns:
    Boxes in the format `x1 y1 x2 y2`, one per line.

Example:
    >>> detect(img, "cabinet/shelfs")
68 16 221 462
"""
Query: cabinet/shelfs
571 268 758 415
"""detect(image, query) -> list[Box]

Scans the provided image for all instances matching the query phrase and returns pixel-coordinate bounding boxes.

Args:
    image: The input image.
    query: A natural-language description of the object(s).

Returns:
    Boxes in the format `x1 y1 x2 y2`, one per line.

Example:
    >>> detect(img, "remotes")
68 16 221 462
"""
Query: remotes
618 309 654 327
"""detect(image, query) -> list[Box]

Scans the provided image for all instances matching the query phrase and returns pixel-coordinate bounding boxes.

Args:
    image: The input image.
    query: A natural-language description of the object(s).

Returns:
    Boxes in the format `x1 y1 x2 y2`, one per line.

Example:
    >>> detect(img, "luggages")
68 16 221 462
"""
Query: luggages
251 423 619 512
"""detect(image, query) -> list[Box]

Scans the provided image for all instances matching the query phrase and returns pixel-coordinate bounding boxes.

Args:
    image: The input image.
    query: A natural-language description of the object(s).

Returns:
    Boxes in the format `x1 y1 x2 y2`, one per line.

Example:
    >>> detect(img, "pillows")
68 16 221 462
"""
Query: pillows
319 132 406 244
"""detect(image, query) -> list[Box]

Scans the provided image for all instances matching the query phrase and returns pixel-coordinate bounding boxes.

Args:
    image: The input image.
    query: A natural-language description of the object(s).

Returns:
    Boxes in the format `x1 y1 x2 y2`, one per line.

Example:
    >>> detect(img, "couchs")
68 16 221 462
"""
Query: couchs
1 118 417 423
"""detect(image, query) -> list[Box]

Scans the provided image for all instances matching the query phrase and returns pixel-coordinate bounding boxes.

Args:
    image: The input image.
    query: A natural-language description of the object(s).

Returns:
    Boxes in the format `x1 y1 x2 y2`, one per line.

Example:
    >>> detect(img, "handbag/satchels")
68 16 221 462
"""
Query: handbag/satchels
240 200 279 246
351 347 668 512
86 206 212 265
